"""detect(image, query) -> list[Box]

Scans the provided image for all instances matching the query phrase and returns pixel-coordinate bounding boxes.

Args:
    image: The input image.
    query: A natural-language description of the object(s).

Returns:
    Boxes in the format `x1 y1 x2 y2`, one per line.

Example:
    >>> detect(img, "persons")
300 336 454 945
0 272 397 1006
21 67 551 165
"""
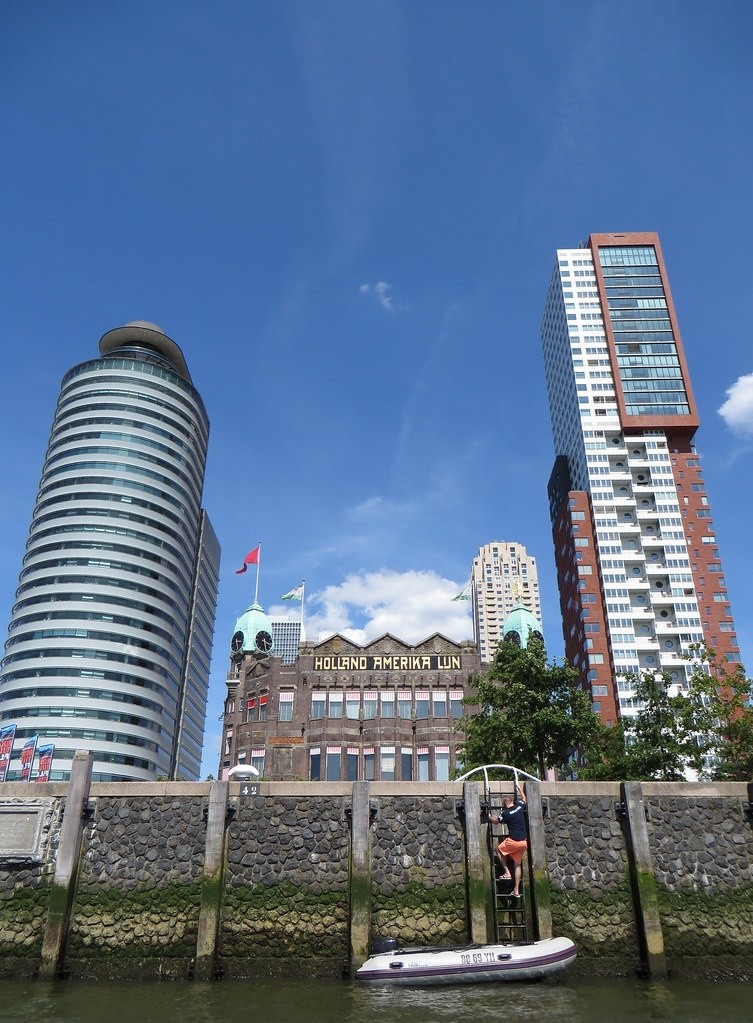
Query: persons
487 782 528 898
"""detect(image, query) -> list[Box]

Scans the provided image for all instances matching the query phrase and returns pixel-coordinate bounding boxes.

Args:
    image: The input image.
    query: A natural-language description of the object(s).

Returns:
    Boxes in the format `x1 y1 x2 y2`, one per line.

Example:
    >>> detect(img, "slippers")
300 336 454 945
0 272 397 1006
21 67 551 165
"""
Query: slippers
499 875 512 880
511 891 520 897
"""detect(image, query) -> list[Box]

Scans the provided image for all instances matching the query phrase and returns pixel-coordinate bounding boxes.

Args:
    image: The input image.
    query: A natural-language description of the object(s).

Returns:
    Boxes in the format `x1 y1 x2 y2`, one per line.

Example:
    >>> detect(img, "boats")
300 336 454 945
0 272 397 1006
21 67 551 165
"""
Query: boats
356 935 578 986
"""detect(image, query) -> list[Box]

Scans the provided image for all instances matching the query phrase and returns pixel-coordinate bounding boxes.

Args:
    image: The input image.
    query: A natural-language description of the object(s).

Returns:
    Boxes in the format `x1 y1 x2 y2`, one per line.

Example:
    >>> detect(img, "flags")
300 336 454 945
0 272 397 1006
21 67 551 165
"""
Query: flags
20 734 39 782
450 582 473 602
236 546 259 574
0 723 17 782
35 742 56 781
280 583 303 601
